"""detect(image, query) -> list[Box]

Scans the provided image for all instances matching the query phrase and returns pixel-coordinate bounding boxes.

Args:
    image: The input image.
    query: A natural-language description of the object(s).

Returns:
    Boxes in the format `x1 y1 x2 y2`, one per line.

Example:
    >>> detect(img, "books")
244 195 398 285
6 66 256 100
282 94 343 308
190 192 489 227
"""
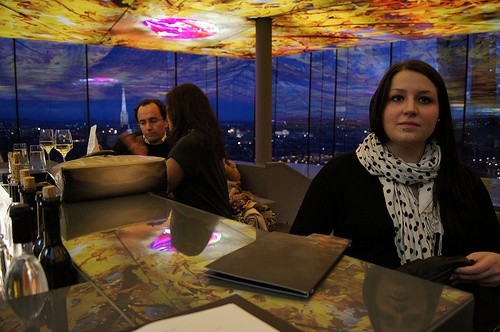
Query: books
202 231 354 299
126 293 305 331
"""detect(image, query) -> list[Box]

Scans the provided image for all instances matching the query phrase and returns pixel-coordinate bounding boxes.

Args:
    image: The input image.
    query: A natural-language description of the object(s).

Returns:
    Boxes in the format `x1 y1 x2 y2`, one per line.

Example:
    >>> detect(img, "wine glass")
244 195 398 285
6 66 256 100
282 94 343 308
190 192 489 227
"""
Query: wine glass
54 129 73 162
39 129 54 164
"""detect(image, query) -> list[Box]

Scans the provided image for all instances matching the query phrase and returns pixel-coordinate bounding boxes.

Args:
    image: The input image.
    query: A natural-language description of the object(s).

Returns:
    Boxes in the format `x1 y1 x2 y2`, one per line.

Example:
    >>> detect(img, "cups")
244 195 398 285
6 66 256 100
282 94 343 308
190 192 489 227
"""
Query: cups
30 145 43 170
13 143 27 163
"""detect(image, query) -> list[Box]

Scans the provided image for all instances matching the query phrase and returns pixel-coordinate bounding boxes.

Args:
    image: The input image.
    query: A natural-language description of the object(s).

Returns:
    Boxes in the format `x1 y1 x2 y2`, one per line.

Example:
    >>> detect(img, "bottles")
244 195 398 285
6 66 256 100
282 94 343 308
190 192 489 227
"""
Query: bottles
0 152 74 301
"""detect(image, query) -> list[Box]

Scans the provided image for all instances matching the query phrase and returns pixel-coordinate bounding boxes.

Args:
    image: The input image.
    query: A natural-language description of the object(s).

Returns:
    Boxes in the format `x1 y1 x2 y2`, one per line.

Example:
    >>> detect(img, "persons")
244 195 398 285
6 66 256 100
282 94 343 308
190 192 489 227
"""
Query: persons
108 98 178 161
168 200 222 259
288 59 500 332
121 83 241 219
359 260 445 331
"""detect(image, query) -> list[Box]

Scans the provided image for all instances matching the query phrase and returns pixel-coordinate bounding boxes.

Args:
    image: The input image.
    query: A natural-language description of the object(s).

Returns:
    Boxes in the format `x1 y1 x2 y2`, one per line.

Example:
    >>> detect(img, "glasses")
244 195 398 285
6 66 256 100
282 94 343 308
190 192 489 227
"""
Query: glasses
136 118 166 124
